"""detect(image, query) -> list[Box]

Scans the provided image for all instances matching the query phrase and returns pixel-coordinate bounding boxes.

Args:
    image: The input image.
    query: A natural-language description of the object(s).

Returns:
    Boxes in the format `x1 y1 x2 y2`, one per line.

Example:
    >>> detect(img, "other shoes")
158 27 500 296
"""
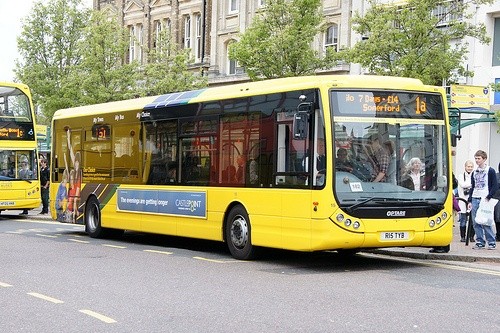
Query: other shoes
429 249 449 253
19 211 28 216
39 211 48 214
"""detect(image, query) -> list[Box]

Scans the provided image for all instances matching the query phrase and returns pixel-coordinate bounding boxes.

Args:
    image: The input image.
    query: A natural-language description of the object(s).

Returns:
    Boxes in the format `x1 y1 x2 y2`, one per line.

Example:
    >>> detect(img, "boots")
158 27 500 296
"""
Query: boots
470 225 475 242
459 226 465 242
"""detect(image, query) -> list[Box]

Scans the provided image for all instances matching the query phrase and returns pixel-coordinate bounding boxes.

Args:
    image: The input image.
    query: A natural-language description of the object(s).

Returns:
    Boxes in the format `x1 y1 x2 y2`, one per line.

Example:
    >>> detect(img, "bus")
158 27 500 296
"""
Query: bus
0 82 42 215
49 74 455 262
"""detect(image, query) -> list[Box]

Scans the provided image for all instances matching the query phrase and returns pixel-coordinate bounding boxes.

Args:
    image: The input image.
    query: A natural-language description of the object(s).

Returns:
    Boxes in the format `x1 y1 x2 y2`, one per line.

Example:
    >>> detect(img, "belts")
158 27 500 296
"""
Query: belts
474 197 481 199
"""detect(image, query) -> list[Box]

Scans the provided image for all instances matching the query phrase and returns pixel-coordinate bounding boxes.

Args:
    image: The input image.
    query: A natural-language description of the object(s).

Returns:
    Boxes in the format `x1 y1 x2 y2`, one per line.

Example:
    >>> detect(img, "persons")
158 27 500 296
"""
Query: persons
429 170 458 254
474 187 499 251
494 163 500 243
55 128 83 223
457 159 476 243
467 149 500 250
37 159 50 215
150 117 427 192
19 161 34 216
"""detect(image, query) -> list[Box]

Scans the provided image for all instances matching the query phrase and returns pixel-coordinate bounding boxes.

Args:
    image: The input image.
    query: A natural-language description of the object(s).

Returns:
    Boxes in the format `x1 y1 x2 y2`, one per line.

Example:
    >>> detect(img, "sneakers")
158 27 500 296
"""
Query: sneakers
488 243 496 250
472 242 485 249
